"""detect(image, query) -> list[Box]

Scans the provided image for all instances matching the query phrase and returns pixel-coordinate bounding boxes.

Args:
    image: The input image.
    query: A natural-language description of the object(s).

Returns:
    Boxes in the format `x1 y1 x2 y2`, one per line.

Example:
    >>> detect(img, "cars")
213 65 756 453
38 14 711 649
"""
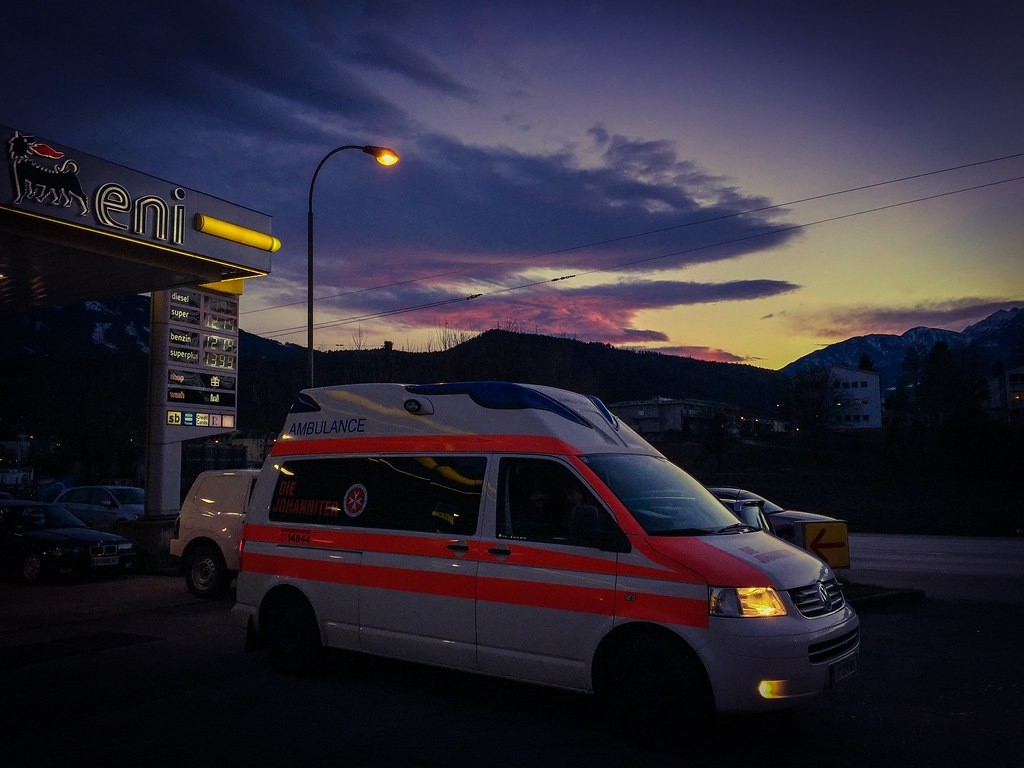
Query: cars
638 486 835 549
0 484 147 587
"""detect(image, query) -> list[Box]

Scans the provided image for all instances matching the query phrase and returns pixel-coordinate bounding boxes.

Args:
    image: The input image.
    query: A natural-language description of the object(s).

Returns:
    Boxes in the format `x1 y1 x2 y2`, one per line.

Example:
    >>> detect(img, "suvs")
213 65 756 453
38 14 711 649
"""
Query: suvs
169 468 261 598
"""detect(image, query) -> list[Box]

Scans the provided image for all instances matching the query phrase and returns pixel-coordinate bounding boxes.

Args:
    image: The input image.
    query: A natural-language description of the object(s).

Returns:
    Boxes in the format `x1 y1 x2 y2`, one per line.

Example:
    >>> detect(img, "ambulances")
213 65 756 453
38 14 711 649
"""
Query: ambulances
231 382 864 752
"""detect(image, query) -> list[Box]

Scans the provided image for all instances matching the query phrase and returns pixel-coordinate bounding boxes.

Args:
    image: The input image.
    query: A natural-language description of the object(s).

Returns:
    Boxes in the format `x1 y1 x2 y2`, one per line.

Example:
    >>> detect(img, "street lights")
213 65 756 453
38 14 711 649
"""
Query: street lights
306 143 401 389
